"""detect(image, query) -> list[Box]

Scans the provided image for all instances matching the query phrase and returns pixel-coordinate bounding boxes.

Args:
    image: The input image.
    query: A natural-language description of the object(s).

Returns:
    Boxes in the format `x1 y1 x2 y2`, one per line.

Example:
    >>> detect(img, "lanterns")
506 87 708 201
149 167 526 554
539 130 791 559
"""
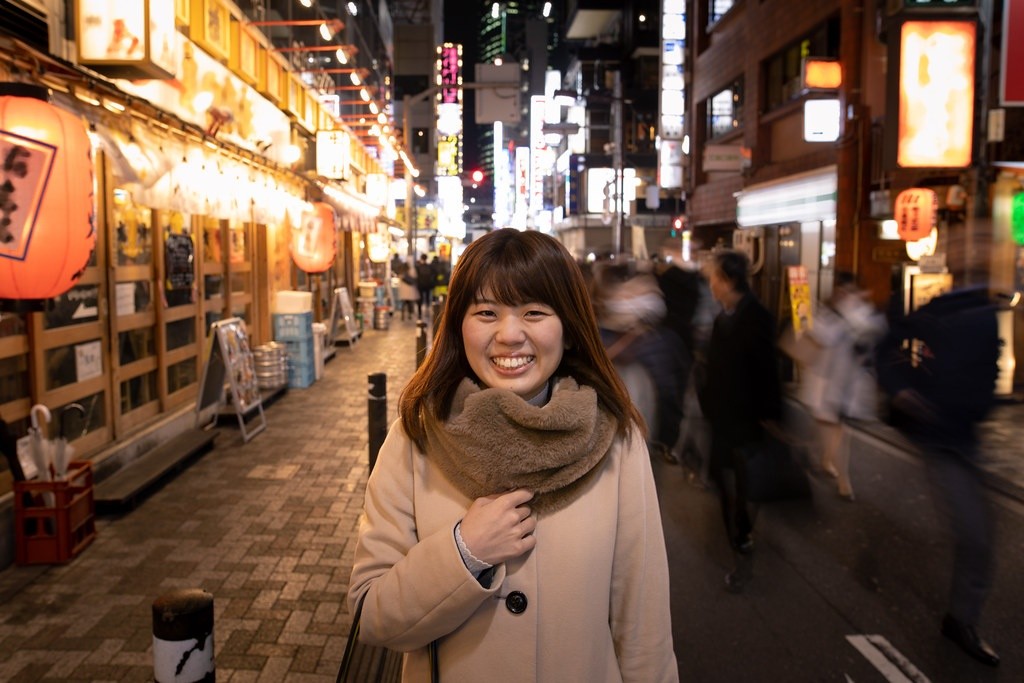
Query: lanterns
894 185 937 242
0 81 97 316
291 197 336 276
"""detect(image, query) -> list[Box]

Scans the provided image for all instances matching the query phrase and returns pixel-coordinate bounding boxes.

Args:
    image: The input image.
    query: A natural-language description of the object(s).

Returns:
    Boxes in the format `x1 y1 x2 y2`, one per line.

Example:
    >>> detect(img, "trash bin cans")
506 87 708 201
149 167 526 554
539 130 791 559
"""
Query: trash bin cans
375 306 390 330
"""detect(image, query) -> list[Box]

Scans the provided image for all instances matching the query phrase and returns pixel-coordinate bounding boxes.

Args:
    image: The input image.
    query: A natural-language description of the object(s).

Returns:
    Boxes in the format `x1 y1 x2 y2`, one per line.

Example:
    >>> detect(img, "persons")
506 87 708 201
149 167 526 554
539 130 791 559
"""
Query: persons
391 253 441 321
588 249 1000 668
348 228 678 683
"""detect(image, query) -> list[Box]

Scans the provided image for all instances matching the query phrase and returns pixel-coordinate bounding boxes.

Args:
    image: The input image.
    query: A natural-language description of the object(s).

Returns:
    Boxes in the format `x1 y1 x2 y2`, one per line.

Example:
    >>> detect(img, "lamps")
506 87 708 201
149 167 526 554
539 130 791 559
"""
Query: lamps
272 44 360 65
282 67 427 196
205 105 234 139
246 18 347 42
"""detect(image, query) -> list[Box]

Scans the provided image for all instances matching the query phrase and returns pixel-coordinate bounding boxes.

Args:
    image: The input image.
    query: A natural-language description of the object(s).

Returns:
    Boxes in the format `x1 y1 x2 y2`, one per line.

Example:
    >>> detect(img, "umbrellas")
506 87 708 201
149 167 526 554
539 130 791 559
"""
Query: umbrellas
0 401 84 507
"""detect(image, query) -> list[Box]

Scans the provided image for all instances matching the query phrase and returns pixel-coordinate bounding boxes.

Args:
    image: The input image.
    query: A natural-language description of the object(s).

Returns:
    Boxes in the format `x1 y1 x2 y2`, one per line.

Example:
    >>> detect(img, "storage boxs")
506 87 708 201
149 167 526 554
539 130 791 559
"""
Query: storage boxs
357 281 378 298
10 459 99 567
271 290 327 390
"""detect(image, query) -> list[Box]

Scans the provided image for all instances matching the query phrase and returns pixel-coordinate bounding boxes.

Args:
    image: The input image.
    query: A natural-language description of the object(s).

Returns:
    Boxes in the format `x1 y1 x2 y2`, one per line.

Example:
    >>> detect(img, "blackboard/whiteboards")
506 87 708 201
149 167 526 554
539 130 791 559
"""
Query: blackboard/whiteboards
328 287 359 341
